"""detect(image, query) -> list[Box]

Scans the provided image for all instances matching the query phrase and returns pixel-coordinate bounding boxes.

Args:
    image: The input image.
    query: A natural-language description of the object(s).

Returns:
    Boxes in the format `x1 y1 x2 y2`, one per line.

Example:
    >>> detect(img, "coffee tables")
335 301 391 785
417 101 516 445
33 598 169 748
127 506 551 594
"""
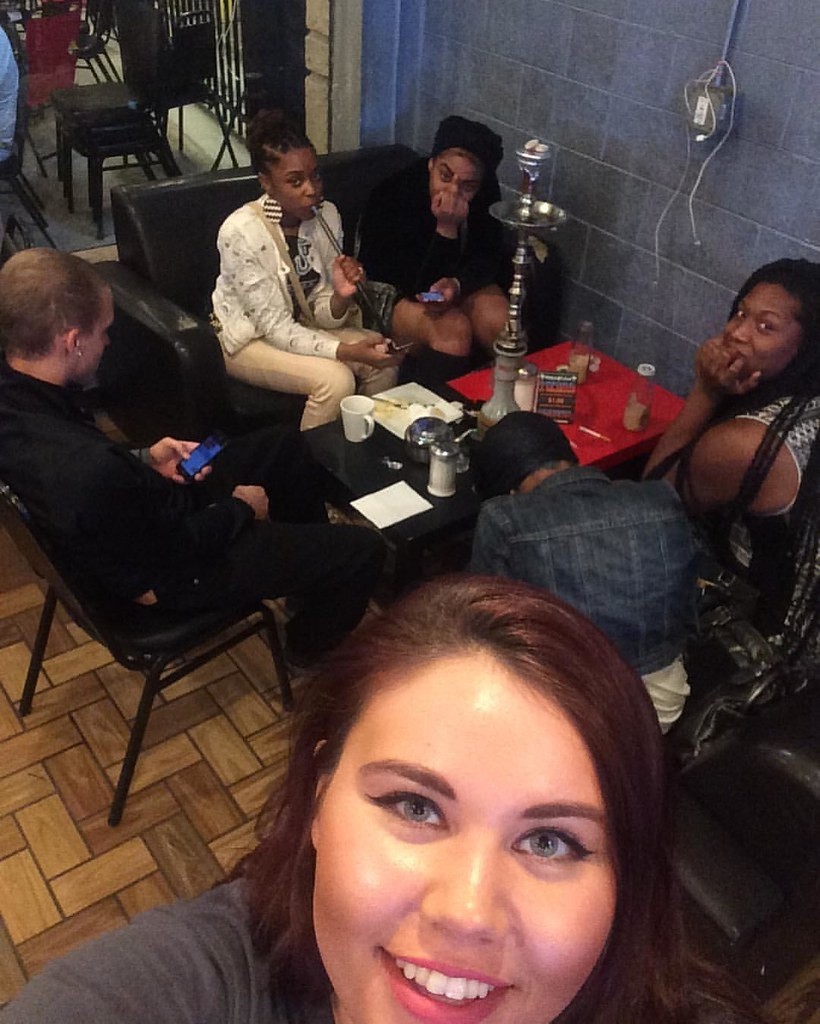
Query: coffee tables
299 381 514 580
447 337 685 518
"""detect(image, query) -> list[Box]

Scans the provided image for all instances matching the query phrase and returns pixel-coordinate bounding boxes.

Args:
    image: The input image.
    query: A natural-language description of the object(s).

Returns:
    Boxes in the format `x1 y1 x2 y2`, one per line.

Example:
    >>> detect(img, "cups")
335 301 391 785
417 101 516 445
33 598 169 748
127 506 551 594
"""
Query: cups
339 394 377 443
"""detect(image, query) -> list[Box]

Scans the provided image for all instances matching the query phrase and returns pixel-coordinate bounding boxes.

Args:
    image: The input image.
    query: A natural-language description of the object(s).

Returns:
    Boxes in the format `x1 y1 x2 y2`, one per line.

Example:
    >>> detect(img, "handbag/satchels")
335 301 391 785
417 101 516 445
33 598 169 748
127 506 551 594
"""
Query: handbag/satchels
674 562 820 753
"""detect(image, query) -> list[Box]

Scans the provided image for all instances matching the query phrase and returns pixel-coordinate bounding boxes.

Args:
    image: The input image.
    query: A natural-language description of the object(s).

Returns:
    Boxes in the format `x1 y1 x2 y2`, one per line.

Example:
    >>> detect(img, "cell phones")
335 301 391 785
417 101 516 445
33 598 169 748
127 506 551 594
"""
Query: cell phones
176 427 234 482
387 343 414 355
420 292 444 303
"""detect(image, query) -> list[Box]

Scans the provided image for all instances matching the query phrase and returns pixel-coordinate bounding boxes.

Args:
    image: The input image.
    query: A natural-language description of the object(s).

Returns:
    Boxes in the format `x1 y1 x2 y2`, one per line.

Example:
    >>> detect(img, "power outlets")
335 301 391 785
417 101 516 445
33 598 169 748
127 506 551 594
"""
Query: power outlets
683 80 742 137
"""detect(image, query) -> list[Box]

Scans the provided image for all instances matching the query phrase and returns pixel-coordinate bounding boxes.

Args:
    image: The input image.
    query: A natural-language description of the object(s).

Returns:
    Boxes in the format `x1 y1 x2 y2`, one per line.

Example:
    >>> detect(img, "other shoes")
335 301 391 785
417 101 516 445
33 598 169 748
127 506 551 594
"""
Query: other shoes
284 629 339 678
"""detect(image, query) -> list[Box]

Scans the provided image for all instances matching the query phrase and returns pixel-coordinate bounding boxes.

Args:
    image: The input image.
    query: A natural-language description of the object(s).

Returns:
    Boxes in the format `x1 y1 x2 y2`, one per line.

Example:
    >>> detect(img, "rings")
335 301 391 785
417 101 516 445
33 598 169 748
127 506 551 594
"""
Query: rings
358 267 363 275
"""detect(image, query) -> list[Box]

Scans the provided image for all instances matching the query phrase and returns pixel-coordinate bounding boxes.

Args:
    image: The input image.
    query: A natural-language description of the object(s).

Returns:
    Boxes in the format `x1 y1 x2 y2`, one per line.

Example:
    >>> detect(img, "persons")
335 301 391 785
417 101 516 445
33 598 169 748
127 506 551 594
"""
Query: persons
471 412 692 737
0 246 388 678
359 115 511 379
644 256 820 700
208 109 407 431
0 576 771 1024
0 27 19 162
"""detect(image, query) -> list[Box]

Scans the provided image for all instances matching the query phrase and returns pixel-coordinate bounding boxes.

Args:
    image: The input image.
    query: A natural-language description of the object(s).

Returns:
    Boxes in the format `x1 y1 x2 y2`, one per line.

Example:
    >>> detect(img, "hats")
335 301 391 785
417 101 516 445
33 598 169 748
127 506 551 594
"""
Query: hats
477 410 580 494
432 115 505 169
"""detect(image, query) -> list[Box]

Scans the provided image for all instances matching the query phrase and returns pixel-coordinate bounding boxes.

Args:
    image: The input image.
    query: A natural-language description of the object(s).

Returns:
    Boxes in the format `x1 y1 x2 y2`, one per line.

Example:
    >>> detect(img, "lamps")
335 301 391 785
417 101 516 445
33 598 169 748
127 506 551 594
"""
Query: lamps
512 139 556 219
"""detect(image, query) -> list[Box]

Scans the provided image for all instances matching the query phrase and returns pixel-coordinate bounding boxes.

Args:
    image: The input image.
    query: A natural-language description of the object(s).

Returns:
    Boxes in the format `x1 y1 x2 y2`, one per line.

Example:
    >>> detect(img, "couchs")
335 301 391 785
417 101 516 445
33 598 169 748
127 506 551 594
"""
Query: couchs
608 675 820 976
93 145 572 447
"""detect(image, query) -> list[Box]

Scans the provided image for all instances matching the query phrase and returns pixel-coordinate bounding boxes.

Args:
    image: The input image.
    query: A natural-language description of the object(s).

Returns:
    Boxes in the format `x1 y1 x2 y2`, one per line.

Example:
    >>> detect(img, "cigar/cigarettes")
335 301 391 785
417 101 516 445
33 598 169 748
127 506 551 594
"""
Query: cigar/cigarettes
579 426 611 443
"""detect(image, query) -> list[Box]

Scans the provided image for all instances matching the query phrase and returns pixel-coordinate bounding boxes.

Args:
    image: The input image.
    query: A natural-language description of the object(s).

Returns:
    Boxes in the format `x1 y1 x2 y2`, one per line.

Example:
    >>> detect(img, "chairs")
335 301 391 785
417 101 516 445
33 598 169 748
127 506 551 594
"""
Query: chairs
0 478 297 828
0 1 255 251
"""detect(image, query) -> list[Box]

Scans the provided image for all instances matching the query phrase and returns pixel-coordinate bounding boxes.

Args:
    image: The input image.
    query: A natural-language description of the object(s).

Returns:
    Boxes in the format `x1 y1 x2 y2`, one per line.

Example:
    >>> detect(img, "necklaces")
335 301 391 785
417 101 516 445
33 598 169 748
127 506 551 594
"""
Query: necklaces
282 224 299 228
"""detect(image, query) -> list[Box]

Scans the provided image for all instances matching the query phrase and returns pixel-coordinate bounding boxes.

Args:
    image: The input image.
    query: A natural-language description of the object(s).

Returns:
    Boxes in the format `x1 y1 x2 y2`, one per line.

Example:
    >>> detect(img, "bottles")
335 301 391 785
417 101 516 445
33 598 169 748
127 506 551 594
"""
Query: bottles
623 363 656 432
568 321 594 384
514 360 539 412
426 441 460 498
